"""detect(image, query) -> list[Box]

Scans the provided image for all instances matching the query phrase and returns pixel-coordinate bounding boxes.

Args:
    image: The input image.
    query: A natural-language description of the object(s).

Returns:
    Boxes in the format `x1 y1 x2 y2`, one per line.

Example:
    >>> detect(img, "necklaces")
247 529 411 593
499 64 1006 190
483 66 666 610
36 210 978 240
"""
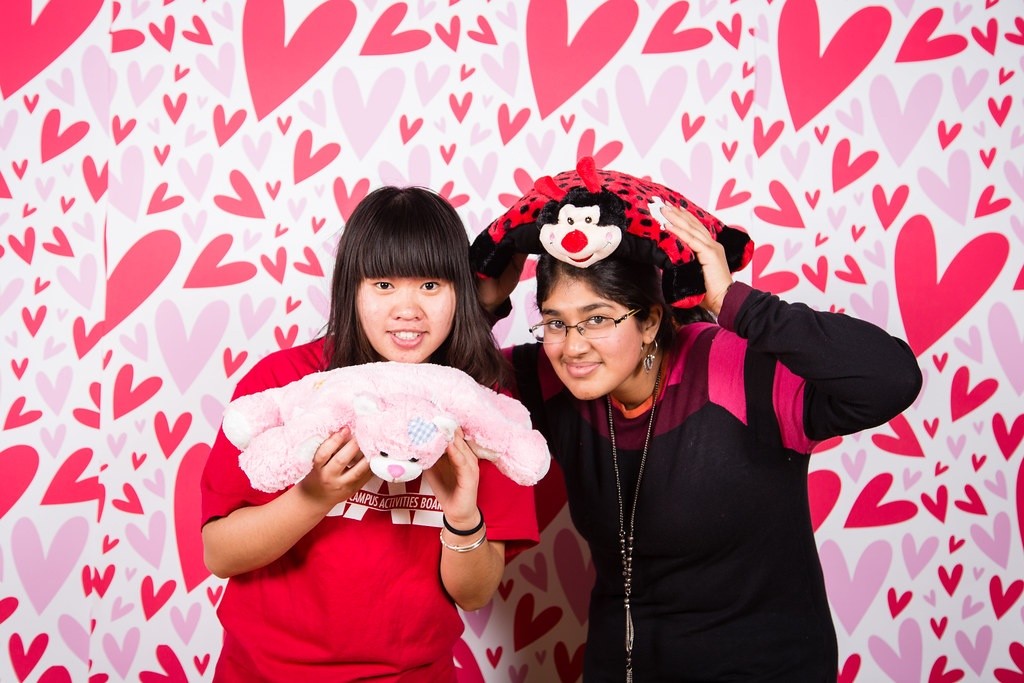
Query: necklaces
608 359 661 683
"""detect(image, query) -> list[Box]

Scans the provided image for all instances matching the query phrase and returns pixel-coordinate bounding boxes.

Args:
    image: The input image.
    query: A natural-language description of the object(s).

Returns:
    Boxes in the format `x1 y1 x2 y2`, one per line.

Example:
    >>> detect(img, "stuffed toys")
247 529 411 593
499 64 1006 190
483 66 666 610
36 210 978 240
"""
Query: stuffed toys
471 156 755 309
222 361 551 493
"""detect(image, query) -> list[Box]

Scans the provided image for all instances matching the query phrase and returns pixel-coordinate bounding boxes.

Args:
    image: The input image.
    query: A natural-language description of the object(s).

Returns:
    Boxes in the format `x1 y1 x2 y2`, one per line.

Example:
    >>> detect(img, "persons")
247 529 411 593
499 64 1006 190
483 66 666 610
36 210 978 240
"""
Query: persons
201 185 540 683
477 204 923 683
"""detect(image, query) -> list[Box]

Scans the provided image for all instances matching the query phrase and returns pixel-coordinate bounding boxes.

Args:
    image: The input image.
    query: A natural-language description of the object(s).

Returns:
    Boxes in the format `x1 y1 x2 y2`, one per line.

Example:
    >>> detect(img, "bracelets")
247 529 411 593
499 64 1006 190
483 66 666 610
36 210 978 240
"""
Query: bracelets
443 509 484 536
440 524 487 553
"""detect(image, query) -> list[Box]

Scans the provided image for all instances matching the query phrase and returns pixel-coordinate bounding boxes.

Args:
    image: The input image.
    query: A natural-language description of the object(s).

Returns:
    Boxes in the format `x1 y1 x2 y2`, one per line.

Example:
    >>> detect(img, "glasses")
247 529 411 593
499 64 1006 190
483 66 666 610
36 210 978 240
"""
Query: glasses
529 308 640 343
